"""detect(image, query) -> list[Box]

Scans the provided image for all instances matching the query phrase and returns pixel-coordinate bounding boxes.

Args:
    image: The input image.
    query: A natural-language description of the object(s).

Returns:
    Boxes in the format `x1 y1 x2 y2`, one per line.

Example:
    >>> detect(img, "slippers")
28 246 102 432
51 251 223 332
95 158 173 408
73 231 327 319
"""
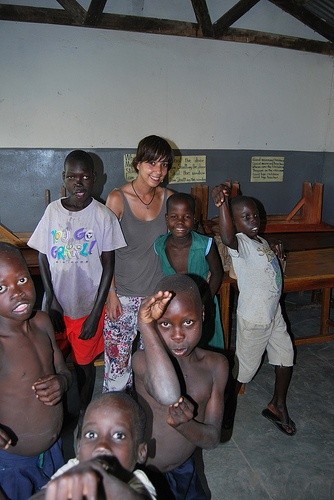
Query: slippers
261 408 297 437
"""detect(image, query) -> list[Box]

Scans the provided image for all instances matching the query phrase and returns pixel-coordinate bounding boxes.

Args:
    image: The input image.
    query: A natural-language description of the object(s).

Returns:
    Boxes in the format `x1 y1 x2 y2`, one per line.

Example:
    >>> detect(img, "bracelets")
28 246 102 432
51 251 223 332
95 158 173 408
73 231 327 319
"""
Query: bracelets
110 287 117 291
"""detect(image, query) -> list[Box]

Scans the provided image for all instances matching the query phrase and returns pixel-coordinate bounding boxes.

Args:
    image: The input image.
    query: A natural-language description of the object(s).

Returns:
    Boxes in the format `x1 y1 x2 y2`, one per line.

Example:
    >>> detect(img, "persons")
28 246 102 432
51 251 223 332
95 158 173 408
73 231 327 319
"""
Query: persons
103 135 180 394
151 193 226 355
26 149 128 442
0 242 232 500
213 184 297 443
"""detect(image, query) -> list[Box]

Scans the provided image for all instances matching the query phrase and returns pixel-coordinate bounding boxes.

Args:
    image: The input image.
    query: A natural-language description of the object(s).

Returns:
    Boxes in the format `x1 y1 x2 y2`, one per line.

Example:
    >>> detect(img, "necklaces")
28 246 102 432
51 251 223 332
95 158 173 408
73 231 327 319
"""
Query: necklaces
130 180 157 210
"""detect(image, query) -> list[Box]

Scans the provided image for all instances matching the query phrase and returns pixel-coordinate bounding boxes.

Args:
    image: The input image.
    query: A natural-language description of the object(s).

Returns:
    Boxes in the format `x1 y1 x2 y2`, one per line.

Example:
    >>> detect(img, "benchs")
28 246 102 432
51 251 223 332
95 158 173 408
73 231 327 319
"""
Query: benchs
0 187 105 367
190 178 334 396
